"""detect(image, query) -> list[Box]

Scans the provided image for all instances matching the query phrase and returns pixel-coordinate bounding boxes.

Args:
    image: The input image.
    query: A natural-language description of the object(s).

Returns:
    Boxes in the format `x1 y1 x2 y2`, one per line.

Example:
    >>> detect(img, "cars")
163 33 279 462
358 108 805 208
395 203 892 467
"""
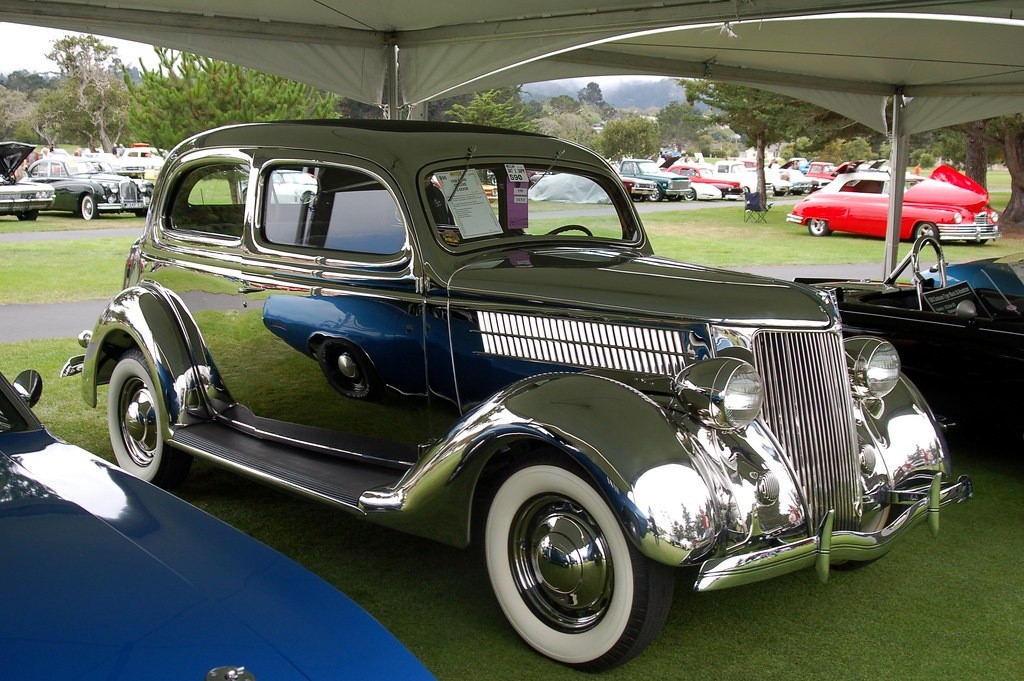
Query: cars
1 371 431 681
237 168 317 205
38 142 169 171
60 120 972 673
429 175 499 205
786 168 1003 247
0 140 57 220
23 156 155 222
802 235 1024 456
531 153 890 203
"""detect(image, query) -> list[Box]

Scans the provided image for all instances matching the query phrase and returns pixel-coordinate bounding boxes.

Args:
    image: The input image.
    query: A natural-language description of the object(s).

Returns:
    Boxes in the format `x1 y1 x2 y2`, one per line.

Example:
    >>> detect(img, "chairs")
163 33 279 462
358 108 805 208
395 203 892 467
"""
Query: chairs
744 192 771 223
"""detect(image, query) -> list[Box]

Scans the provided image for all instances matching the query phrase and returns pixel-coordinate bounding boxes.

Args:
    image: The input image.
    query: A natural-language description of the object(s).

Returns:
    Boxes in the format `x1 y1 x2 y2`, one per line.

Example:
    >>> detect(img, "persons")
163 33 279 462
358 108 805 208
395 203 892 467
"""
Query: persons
914 162 921 175
792 161 799 170
112 144 125 156
25 150 39 169
768 158 776 168
41 148 52 159
